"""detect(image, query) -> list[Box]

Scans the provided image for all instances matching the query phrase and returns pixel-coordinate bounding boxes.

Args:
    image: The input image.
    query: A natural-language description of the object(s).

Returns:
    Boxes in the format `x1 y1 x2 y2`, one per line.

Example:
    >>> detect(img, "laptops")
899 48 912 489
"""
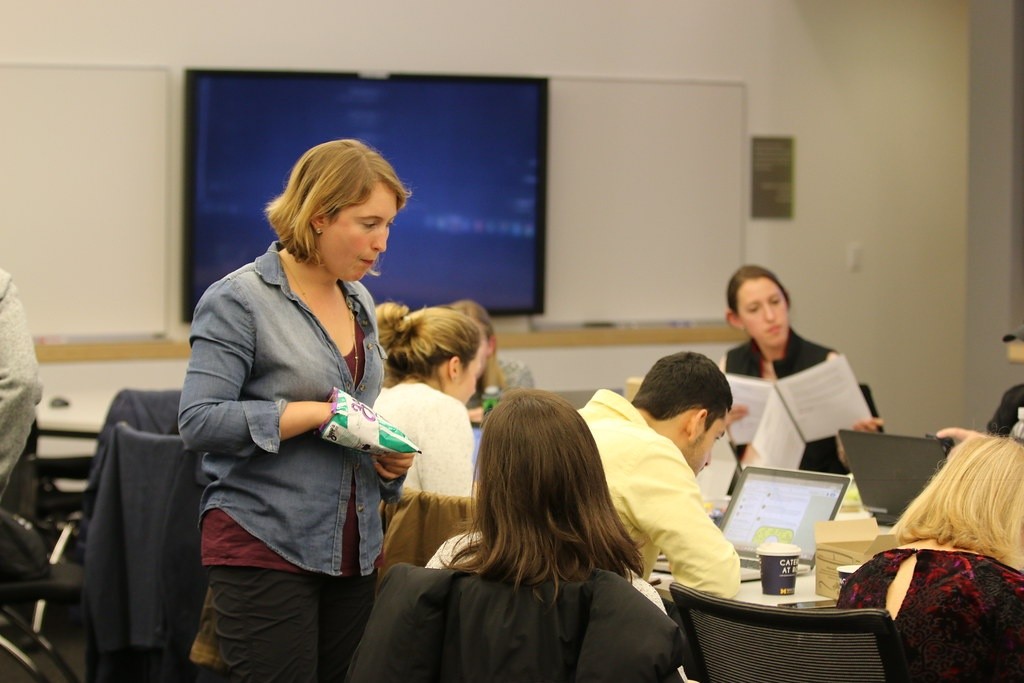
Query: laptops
838 429 948 526
653 465 852 582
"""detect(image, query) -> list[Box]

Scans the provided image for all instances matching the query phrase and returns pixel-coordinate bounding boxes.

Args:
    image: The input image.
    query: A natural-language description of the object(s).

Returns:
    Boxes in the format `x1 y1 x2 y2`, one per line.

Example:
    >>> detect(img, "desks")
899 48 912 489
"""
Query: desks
648 564 838 611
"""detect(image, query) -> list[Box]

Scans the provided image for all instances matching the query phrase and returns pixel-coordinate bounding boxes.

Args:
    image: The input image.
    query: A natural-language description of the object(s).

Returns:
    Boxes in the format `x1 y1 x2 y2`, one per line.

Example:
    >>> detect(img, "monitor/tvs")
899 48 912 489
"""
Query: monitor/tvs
180 67 546 326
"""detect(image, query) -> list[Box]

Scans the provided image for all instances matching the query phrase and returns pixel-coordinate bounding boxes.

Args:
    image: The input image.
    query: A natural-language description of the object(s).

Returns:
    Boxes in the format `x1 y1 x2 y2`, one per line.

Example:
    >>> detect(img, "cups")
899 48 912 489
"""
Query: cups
757 543 802 595
837 565 862 595
721 497 731 516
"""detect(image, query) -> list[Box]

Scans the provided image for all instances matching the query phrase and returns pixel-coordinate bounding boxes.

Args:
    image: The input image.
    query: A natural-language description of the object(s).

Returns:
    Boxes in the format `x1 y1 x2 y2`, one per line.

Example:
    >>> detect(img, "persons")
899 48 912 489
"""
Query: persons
425 388 690 683
726 259 885 475
836 328 1023 681
573 351 741 603
0 269 43 503
368 300 530 502
178 140 414 682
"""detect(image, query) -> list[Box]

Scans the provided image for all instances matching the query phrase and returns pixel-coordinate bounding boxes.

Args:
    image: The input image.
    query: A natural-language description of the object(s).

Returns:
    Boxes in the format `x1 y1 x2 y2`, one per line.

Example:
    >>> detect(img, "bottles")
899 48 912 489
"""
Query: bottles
1010 407 1024 446
482 386 501 417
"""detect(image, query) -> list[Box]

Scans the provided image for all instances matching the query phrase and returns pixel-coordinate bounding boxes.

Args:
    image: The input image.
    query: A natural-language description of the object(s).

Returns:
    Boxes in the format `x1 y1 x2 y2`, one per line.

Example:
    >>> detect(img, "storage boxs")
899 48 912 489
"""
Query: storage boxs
815 517 899 600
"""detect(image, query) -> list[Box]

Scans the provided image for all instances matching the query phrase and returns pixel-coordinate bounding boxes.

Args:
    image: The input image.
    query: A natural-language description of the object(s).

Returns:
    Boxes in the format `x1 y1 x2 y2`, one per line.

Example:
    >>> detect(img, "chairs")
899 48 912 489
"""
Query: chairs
0 424 102 683
670 581 912 683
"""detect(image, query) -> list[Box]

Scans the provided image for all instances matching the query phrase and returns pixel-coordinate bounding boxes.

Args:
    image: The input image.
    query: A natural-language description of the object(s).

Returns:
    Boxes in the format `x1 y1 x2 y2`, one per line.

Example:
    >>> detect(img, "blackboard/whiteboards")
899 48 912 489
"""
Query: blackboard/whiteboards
0 62 169 338
527 77 751 330
